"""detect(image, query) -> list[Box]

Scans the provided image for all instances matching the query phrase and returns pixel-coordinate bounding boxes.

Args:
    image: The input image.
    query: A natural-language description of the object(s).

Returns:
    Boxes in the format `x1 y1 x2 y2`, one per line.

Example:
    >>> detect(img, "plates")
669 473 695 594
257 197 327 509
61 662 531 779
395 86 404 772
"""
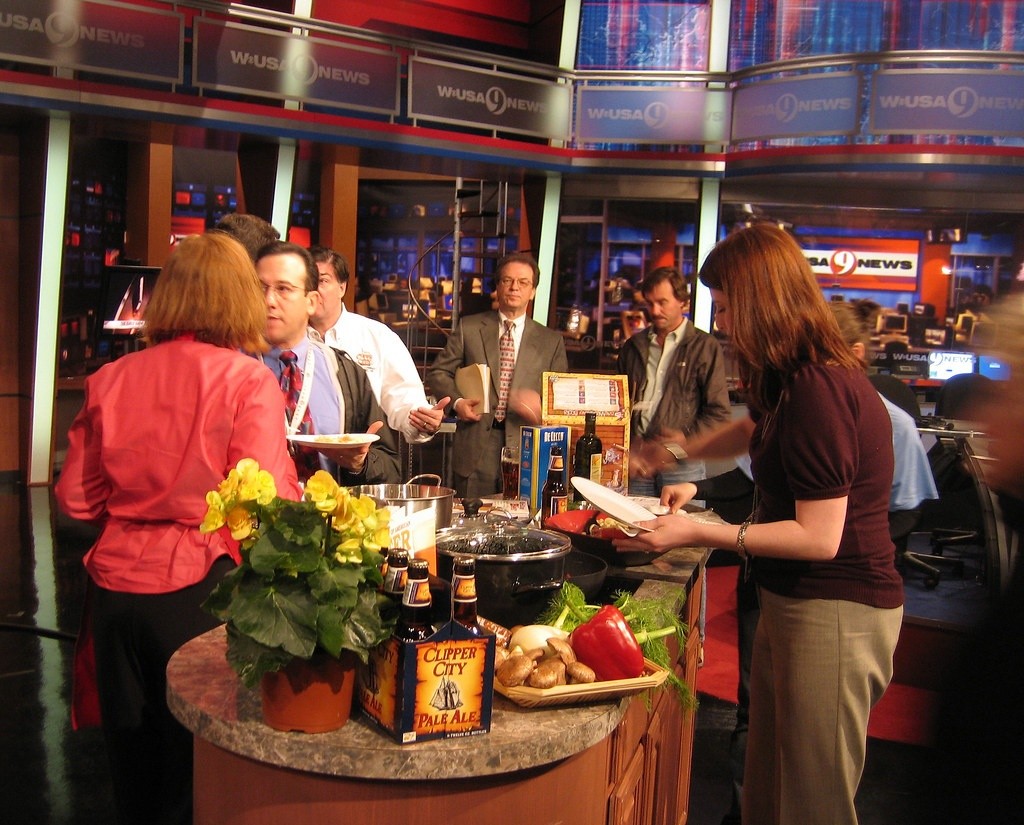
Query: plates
286 434 380 448
571 476 658 532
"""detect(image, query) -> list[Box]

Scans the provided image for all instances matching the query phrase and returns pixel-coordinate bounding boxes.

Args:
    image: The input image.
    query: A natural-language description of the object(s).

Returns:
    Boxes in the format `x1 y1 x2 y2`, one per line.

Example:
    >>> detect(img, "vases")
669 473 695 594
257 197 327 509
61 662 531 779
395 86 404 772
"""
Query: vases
265 650 355 735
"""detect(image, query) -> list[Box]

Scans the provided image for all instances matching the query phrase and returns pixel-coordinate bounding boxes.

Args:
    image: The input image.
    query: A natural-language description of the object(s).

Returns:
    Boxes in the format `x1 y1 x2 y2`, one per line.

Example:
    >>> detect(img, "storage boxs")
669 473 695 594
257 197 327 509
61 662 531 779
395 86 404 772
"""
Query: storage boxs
516 426 571 518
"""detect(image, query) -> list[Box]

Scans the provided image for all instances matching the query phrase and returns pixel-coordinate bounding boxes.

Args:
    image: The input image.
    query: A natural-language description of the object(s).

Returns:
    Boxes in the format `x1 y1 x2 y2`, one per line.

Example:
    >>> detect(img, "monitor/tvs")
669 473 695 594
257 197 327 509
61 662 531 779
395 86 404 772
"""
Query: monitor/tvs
865 313 1010 381
719 340 740 381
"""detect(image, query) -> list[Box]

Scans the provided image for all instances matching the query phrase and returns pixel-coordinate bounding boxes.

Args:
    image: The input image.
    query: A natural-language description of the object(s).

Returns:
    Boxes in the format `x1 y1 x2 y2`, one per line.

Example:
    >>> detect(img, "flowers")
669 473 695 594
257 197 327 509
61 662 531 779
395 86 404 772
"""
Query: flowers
200 458 398 685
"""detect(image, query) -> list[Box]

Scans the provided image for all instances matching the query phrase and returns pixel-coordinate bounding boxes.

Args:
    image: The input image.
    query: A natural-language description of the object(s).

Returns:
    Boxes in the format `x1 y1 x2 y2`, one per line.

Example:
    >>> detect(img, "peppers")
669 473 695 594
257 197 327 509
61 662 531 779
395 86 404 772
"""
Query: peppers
564 599 645 680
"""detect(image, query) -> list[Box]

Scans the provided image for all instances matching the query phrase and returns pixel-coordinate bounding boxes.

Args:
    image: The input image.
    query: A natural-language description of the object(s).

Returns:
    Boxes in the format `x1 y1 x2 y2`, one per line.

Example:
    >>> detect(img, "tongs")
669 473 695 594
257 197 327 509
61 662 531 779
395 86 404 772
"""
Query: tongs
934 528 977 541
903 549 967 576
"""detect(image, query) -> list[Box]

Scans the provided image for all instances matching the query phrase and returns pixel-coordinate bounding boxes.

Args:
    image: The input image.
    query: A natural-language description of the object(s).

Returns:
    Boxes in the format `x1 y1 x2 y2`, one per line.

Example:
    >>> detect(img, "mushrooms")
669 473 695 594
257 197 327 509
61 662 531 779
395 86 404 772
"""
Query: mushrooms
496 637 596 689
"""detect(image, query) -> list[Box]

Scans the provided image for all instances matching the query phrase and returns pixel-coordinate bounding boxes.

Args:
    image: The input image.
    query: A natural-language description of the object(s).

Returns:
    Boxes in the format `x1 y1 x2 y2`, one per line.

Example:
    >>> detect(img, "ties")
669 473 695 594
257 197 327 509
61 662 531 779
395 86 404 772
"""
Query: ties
495 320 517 422
278 348 320 483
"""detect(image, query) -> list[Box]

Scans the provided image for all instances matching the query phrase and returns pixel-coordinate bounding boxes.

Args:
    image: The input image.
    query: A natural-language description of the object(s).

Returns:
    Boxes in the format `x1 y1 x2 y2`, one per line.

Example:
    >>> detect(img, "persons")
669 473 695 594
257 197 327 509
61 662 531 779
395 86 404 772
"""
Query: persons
616 265 733 509
217 207 282 264
52 229 305 825
253 240 401 490
611 226 904 825
306 245 451 445
628 298 940 788
427 250 569 498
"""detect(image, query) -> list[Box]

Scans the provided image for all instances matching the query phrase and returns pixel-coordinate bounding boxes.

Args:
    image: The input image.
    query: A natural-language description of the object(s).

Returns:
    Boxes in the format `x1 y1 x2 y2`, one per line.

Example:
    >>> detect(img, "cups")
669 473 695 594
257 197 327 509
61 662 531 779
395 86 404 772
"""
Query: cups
501 446 519 501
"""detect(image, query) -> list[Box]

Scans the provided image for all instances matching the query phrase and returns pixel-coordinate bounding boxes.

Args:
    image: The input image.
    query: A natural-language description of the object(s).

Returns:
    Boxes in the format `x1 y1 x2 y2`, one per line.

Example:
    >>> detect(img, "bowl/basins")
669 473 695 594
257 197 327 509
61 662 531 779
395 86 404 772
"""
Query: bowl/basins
544 510 673 566
564 550 608 592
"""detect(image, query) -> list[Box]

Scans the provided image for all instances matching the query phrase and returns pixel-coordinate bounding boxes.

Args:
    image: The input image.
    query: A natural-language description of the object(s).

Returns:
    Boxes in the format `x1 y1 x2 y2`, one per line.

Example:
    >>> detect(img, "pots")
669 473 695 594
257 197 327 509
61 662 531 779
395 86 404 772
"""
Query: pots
436 507 571 629
347 474 457 535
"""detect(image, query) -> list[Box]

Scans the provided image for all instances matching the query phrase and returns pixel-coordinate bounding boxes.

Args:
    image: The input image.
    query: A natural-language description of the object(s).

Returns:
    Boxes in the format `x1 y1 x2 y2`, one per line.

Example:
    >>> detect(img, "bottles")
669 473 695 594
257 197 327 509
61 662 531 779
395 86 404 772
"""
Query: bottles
394 558 434 641
378 547 409 636
573 413 602 503
542 447 568 530
452 555 485 637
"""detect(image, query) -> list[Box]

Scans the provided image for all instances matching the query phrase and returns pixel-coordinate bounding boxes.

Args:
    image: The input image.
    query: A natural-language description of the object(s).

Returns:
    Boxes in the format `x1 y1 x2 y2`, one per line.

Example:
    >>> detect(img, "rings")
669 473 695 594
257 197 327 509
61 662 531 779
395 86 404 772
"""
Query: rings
641 467 647 474
424 423 426 426
645 550 649 554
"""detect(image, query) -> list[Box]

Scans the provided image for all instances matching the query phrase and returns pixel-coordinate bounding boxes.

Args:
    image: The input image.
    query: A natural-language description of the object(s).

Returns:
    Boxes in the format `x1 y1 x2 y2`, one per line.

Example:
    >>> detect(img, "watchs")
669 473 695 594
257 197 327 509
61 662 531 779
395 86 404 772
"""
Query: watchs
666 443 688 463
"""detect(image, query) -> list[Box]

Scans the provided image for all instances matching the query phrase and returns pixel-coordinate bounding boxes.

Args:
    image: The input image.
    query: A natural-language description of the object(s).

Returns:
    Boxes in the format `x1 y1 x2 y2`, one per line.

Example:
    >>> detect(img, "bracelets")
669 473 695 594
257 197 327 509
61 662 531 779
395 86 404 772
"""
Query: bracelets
737 521 749 560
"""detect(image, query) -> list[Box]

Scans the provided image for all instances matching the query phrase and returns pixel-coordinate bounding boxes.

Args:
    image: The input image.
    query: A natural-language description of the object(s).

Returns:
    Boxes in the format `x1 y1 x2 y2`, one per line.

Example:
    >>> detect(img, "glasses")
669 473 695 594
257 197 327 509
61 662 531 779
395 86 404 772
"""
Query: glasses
499 277 533 289
260 282 308 299
